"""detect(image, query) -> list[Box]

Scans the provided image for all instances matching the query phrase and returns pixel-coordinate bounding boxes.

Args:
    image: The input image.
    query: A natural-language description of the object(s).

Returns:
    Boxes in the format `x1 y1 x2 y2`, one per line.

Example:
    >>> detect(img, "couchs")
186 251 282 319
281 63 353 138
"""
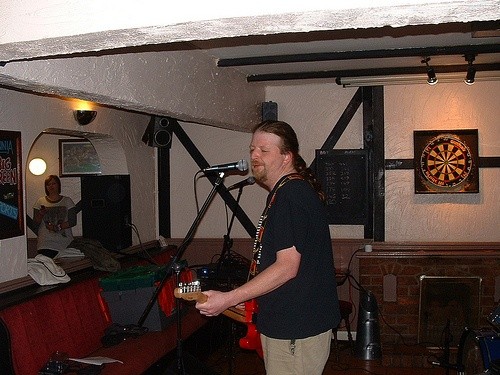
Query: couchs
0 244 226 375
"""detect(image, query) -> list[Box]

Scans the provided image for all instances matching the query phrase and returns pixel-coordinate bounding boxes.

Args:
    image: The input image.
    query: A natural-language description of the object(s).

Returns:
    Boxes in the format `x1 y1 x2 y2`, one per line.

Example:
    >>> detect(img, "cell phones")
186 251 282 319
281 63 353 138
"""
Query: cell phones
41 205 48 214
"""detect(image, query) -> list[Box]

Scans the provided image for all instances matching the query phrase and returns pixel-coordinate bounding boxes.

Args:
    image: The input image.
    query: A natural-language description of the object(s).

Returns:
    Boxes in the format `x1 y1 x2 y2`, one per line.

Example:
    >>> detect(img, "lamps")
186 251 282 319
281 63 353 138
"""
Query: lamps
420 54 439 86
75 109 97 126
461 51 478 86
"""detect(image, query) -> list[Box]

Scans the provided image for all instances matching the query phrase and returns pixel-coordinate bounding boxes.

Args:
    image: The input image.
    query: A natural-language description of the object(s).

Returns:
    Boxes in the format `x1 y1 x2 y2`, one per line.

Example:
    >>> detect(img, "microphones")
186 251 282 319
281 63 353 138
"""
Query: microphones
228 176 256 190
203 160 248 172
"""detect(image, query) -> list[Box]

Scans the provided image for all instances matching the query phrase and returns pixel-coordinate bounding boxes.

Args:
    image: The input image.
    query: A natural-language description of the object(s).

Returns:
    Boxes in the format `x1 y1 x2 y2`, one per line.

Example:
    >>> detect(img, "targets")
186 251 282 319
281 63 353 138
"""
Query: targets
420 136 473 188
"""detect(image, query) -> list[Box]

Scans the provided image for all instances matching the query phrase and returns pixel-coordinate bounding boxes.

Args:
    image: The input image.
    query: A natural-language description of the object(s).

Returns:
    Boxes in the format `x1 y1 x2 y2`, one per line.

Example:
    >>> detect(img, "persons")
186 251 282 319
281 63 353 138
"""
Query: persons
32 175 76 259
195 120 339 375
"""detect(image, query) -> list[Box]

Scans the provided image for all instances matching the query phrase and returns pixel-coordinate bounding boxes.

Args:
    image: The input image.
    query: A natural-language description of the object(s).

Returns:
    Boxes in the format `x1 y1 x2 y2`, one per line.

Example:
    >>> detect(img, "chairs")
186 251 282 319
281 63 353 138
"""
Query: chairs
332 300 356 363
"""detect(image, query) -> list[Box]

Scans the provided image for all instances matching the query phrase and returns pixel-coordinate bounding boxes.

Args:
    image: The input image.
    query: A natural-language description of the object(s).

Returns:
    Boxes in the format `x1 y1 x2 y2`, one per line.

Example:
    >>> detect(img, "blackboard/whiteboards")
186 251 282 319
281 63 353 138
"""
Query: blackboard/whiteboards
315 149 368 226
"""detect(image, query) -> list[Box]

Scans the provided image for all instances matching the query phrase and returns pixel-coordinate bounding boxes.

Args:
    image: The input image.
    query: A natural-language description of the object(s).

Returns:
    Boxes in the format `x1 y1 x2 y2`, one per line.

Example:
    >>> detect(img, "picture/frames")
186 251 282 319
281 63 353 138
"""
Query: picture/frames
58 139 101 178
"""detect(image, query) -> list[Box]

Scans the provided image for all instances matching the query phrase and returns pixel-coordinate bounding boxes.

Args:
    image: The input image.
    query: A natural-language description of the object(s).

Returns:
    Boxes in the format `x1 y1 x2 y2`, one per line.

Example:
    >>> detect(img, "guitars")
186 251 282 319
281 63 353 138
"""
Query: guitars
172 280 267 362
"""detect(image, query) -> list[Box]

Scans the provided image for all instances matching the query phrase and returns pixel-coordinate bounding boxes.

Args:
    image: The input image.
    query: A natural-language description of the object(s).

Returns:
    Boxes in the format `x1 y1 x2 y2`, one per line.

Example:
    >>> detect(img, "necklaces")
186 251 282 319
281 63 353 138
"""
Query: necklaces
253 210 265 264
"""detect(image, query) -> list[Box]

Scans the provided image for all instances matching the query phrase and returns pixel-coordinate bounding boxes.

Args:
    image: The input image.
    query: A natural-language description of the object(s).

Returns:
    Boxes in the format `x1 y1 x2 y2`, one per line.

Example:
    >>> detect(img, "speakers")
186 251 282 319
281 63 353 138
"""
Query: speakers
197 267 250 335
81 175 131 253
